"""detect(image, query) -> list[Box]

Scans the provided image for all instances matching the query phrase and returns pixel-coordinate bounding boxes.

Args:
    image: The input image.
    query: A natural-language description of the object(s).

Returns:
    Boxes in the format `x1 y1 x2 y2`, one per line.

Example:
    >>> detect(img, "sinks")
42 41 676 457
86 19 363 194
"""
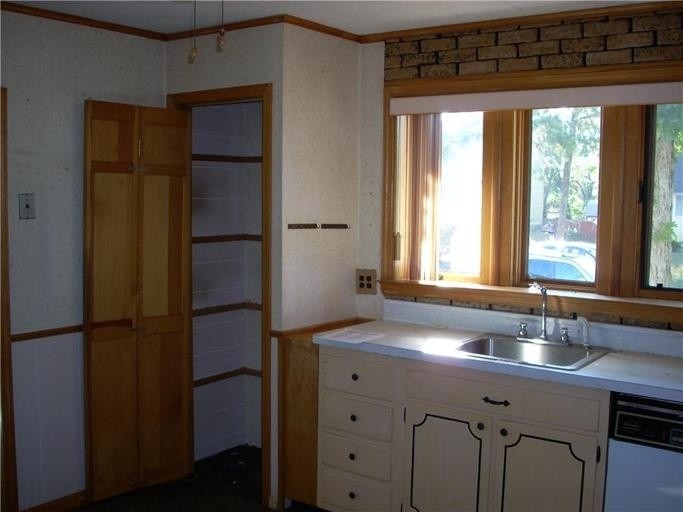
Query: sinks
454 332 611 371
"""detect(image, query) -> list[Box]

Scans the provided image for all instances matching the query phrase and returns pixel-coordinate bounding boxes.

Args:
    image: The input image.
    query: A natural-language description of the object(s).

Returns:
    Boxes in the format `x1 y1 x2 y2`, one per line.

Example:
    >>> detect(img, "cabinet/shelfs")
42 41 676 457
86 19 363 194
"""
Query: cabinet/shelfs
316 344 609 512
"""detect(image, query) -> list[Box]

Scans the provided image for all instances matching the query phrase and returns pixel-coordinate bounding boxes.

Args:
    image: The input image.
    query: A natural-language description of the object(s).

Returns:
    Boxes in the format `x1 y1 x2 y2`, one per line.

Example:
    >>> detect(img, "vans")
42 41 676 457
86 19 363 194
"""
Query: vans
668 191 682 253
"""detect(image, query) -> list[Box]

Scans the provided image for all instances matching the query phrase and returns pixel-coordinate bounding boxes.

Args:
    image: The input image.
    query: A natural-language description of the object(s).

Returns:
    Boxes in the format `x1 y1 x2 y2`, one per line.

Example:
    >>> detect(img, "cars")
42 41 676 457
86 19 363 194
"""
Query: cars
526 215 597 283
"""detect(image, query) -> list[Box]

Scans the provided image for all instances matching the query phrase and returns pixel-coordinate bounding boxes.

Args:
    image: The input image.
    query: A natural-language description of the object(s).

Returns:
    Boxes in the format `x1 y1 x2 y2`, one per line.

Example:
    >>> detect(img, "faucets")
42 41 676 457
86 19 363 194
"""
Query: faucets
570 310 591 349
540 284 548 339
